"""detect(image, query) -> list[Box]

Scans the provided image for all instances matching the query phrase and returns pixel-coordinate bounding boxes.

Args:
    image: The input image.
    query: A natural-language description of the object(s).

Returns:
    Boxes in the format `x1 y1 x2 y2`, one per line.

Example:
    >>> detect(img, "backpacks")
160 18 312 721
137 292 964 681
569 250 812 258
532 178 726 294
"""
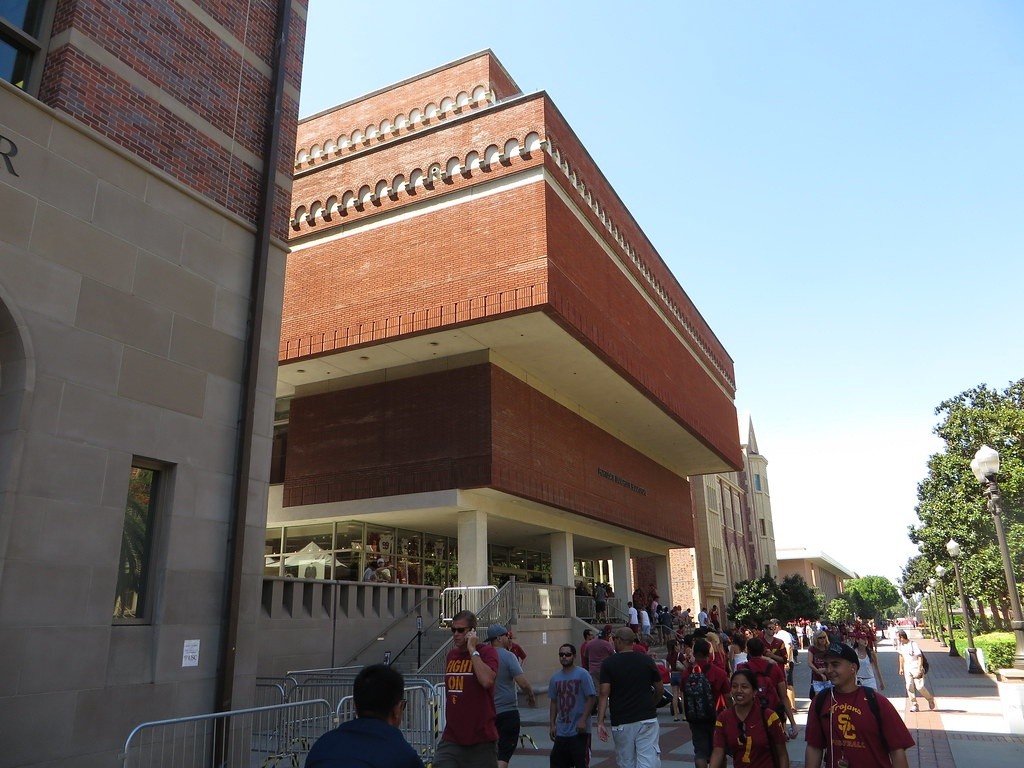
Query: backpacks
685 663 715 723
746 662 784 716
911 641 929 675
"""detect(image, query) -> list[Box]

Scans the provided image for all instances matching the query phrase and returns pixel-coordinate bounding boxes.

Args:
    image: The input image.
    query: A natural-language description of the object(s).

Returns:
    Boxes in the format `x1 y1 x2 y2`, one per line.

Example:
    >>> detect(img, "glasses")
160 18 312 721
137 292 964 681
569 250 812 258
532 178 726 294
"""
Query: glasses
559 653 574 657
738 720 750 742
818 636 826 639
449 627 467 634
767 626 777 631
507 638 512 639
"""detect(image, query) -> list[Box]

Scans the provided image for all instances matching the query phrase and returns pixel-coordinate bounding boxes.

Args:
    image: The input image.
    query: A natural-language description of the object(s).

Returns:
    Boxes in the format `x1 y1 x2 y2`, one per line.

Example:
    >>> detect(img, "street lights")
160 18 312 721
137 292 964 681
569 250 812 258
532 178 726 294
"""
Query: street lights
929 578 947 647
970 444 1024 670
935 565 960 656
924 593 934 639
946 538 985 674
926 586 940 642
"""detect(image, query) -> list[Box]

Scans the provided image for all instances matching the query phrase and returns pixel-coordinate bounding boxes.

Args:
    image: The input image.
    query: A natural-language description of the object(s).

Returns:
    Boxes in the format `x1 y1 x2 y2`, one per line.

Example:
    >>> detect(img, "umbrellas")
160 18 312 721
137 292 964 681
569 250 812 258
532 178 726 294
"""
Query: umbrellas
266 541 347 568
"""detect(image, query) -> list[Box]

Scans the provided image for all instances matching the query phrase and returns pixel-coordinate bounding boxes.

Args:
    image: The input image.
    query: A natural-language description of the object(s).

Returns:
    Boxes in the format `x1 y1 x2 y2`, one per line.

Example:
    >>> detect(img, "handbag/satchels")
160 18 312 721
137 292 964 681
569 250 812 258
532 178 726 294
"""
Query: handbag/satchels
810 679 830 697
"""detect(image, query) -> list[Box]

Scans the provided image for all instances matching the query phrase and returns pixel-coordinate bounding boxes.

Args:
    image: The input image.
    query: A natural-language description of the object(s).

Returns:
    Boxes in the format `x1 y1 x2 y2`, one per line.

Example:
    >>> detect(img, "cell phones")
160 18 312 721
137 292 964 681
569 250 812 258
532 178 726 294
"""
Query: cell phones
472 628 477 635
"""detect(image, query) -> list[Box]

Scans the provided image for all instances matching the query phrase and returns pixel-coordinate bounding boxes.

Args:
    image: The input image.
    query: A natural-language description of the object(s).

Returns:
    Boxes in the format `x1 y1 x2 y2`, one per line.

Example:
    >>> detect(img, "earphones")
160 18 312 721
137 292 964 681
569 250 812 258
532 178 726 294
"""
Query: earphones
851 668 854 671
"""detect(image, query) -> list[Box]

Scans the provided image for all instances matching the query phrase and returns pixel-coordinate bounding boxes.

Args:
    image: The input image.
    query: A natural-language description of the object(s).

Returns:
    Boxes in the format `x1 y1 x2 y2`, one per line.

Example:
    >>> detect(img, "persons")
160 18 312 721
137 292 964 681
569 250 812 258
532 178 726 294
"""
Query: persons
667 619 798 768
362 558 391 582
895 629 935 712
483 624 532 768
304 664 426 768
888 620 900 648
804 642 916 768
432 610 499 768
795 618 885 692
575 580 613 623
506 632 527 714
547 644 596 768
581 625 615 719
598 627 664 768
709 668 789 768
627 583 720 634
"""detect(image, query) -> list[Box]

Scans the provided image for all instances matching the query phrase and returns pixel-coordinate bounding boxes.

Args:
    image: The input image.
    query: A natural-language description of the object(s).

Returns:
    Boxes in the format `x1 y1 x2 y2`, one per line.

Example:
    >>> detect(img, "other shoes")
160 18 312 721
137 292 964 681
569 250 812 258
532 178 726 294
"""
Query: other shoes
673 718 681 722
683 718 687 721
928 700 935 710
909 702 920 713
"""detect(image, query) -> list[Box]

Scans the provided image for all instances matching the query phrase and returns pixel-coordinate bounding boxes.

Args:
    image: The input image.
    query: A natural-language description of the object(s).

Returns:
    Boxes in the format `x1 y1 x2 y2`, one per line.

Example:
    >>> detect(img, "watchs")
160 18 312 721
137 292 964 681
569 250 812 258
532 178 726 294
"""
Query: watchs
471 650 480 656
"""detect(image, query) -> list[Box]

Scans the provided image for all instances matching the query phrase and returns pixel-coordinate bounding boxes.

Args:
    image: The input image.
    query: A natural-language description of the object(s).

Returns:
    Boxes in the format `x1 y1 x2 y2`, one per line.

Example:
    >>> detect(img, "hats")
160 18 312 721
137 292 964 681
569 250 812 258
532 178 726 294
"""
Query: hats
818 641 860 667
376 558 385 564
857 631 871 639
483 624 509 643
599 624 612 638
